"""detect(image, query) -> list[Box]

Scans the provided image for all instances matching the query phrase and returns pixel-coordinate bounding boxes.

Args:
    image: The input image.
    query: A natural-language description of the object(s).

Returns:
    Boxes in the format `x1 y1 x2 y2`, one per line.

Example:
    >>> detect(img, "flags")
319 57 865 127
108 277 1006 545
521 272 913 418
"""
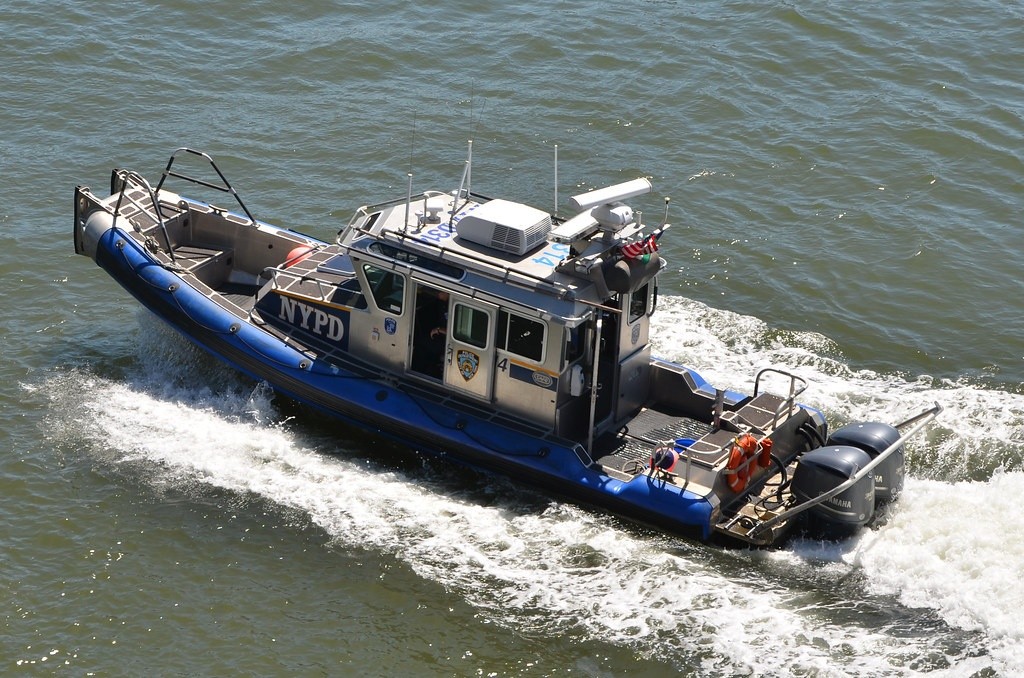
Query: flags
621 234 656 261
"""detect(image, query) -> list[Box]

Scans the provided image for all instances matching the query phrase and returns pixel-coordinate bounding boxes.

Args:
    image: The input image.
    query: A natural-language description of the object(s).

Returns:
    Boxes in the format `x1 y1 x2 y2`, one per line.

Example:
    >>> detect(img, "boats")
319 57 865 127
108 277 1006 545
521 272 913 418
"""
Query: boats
72 139 943 551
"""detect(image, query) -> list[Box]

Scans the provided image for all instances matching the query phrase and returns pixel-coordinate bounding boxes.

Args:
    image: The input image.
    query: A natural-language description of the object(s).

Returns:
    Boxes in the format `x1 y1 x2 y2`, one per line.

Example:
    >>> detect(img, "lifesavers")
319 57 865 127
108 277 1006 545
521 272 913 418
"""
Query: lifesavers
727 436 758 494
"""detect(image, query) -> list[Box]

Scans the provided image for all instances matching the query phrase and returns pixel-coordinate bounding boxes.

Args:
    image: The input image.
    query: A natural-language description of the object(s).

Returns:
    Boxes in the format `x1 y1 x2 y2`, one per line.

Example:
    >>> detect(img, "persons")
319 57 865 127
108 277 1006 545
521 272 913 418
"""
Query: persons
430 292 448 340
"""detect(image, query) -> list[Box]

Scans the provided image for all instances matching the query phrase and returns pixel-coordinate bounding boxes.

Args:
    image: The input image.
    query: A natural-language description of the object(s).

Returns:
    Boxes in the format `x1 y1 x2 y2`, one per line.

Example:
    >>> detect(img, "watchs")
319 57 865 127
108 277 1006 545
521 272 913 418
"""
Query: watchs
437 326 440 333
